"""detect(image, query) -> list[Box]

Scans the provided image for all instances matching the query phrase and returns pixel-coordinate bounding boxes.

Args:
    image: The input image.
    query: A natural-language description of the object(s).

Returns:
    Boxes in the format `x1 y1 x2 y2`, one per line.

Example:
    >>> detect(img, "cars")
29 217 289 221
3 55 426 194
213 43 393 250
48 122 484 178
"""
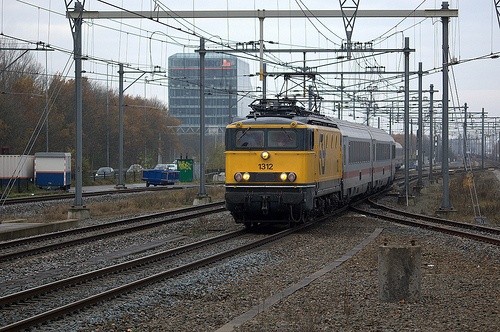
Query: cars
95 167 116 181
125 165 144 180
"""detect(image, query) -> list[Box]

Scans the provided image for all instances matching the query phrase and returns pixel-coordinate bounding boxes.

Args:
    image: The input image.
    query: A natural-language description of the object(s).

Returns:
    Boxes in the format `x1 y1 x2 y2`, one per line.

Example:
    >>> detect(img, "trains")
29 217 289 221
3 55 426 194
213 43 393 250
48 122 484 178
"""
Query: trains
327 120 372 207
225 99 340 228
364 124 394 193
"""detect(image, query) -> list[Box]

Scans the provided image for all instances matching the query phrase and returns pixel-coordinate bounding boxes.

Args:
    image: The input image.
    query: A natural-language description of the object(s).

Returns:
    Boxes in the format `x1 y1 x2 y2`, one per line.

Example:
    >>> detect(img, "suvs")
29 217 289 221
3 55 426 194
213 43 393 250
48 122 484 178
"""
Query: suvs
153 163 177 169
